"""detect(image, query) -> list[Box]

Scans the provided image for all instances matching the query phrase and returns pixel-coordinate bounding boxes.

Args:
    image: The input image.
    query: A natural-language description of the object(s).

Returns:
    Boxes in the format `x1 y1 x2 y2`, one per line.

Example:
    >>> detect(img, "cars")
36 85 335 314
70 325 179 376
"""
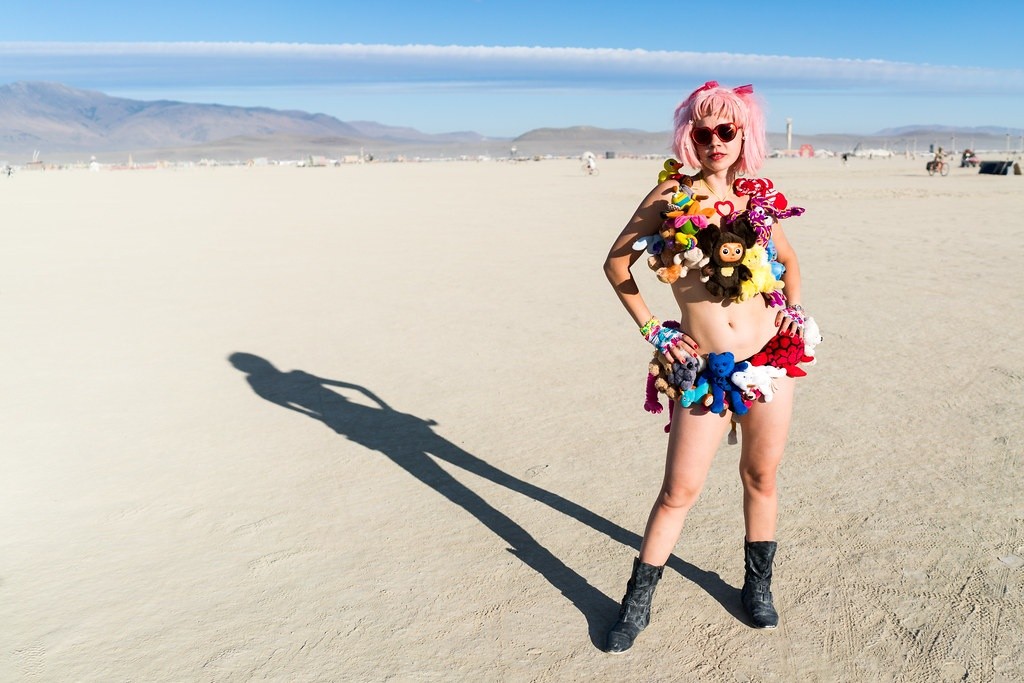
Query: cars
766 152 780 158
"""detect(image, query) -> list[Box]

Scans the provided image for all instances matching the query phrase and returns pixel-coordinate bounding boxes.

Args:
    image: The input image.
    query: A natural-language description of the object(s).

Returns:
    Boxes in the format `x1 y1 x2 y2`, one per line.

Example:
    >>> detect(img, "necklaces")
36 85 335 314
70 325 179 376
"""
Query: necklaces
702 179 735 216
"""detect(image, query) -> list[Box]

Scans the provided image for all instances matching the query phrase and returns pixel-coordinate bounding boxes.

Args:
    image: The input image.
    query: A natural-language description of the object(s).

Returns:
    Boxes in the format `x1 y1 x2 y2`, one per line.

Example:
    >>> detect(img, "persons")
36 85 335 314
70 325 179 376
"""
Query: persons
604 82 804 656
933 146 943 169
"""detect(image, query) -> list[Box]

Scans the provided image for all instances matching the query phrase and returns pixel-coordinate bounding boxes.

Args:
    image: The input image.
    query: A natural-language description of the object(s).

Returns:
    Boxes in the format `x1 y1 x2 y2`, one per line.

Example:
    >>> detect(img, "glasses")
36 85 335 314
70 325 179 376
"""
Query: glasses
691 122 742 146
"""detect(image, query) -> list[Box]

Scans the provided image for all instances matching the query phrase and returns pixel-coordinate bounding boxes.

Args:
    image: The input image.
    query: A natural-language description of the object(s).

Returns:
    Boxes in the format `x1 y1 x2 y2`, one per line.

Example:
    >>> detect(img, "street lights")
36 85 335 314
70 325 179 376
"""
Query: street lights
1006 134 1011 160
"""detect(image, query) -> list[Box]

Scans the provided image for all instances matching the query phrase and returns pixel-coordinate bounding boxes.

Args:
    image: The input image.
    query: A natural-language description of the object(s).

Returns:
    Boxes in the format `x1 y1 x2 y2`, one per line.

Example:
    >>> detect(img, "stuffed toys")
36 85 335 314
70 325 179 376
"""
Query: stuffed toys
632 159 805 305
643 318 823 445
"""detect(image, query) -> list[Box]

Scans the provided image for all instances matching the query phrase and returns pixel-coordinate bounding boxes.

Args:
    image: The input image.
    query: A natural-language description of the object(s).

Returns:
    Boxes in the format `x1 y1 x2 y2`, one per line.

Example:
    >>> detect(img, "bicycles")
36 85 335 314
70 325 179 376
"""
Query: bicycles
928 157 950 176
580 165 599 176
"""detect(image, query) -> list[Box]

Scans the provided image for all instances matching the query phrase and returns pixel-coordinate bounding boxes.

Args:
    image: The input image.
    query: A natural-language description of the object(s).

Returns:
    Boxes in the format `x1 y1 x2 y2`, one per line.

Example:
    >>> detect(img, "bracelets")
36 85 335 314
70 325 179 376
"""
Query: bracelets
793 304 805 314
640 316 659 336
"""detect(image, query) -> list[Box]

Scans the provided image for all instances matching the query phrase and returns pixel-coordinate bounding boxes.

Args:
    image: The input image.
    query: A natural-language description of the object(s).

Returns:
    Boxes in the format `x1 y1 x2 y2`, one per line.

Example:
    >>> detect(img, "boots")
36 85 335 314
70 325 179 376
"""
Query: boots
606 557 663 654
741 535 779 628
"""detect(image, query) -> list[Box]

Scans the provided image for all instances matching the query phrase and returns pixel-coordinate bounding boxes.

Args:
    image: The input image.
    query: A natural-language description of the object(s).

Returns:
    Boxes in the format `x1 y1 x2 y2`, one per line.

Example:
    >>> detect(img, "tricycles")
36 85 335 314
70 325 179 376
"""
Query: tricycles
961 155 976 167
